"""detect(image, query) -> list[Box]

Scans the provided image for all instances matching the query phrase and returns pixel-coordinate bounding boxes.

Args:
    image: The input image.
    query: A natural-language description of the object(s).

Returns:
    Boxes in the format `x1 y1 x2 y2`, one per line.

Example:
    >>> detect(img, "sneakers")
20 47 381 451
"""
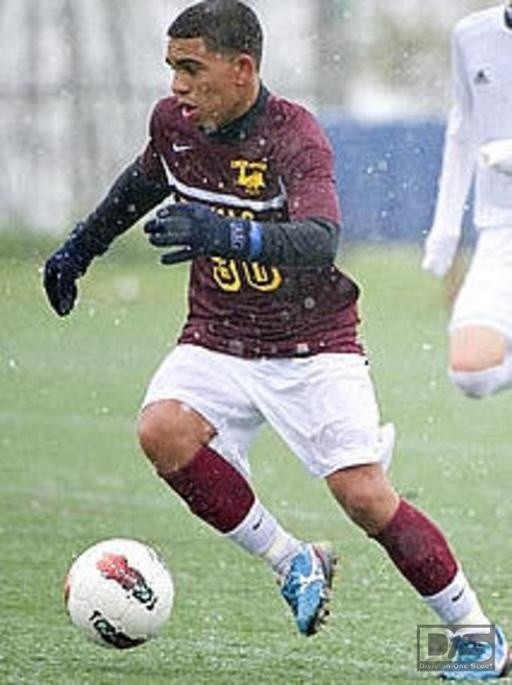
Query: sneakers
437 623 510 681
278 539 338 636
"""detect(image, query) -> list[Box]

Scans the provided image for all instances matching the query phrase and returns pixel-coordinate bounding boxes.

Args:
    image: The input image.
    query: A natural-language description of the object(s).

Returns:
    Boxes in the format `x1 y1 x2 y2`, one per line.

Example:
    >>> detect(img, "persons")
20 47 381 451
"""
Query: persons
40 0 512 681
421 0 512 400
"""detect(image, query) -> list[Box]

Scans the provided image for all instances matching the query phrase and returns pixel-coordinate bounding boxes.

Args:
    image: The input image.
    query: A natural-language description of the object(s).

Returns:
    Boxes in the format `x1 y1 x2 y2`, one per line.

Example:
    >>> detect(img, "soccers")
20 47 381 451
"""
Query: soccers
64 539 174 649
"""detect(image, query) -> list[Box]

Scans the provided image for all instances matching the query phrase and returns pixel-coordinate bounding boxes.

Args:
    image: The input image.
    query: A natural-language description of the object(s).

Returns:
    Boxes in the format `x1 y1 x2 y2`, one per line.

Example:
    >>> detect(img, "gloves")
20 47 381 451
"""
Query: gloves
144 204 254 265
42 219 110 317
